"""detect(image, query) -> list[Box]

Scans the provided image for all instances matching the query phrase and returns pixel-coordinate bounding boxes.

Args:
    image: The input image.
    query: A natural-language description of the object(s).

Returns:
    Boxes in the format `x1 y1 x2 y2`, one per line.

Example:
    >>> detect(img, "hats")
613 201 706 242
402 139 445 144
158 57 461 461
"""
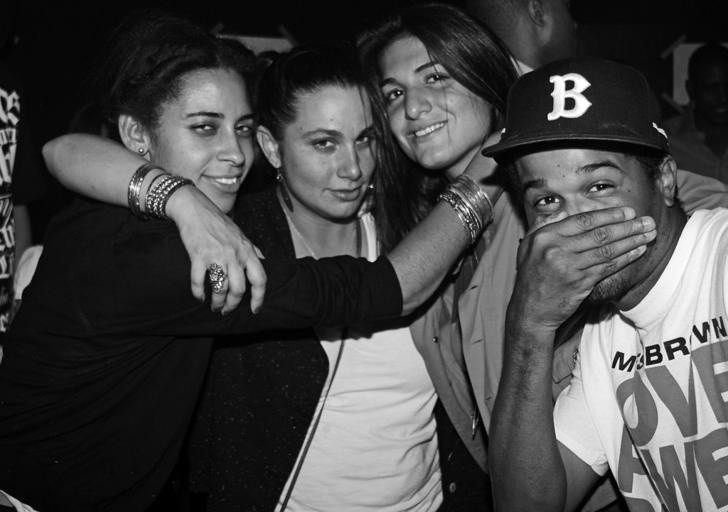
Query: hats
481 55 669 160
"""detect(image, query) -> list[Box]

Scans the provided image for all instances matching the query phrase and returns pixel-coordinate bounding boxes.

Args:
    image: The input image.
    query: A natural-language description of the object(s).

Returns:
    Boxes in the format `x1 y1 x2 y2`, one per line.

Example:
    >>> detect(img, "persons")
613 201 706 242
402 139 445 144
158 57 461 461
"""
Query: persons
0 0 728 511
